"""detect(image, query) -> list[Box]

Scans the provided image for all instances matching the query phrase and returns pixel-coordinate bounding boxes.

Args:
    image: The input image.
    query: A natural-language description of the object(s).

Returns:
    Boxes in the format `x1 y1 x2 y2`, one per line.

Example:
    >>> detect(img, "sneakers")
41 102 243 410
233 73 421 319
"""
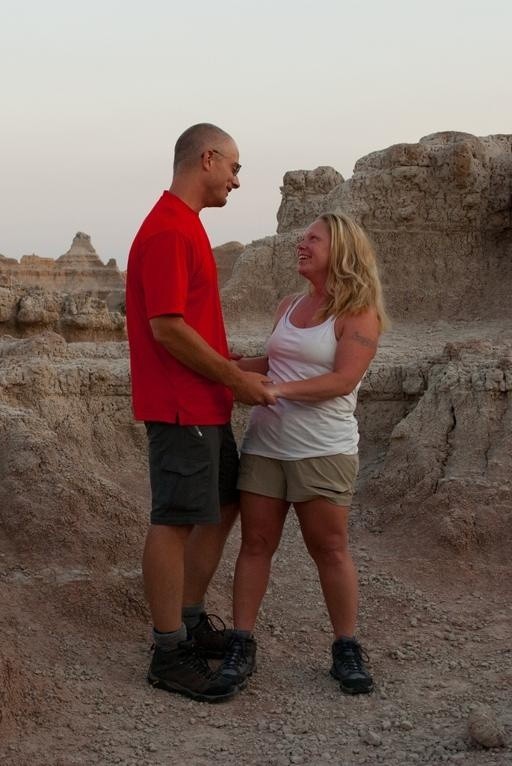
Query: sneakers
330 641 372 694
146 612 258 701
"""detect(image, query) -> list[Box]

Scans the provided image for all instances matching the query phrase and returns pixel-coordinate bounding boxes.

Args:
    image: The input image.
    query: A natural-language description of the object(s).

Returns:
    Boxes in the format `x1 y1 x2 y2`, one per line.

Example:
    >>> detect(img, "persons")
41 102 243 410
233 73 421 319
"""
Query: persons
218 211 394 694
123 123 277 704
